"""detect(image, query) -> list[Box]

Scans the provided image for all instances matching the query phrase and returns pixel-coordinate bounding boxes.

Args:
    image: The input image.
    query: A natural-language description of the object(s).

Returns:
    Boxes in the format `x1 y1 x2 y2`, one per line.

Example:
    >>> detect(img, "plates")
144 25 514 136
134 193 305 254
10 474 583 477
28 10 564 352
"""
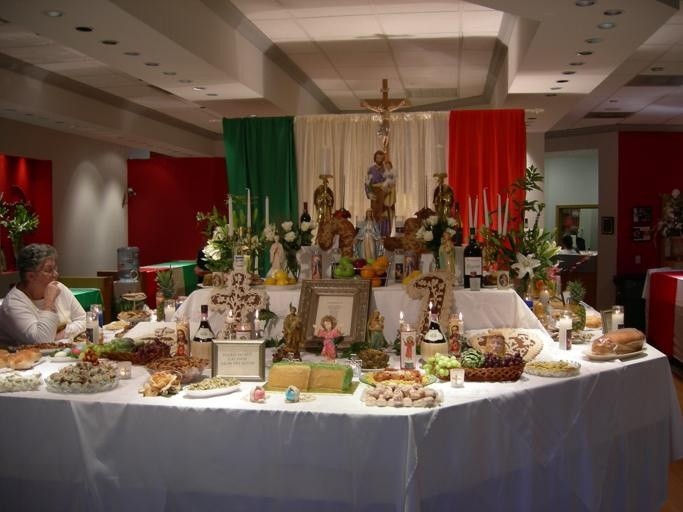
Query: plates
181 382 241 398
354 369 438 390
522 362 582 380
584 346 649 360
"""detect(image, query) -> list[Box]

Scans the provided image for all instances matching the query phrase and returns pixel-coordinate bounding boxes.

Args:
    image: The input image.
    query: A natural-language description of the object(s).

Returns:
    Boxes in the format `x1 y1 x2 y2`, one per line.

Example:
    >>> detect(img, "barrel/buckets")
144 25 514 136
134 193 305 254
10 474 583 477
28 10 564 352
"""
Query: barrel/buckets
117 246 139 280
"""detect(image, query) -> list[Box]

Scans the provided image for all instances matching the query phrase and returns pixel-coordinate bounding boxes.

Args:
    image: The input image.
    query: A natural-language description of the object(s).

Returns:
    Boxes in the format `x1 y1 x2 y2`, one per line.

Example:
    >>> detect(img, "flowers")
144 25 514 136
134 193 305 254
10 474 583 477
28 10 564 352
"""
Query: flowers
654 188 682 238
416 215 458 269
0 192 40 258
264 219 316 277
490 219 562 293
195 204 259 271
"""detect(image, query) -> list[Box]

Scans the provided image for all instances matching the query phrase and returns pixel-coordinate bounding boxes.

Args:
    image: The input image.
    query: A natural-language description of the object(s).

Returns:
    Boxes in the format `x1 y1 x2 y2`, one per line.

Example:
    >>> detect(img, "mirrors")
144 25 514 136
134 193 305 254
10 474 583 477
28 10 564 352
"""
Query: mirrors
557 205 598 272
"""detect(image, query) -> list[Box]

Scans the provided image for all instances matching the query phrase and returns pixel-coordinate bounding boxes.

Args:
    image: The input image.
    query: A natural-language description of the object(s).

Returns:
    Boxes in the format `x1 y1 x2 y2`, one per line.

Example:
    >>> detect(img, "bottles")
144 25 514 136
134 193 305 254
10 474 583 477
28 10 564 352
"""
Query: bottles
310 249 341 279
397 308 464 371
523 285 548 329
190 303 216 361
462 227 483 290
85 303 104 347
298 201 311 246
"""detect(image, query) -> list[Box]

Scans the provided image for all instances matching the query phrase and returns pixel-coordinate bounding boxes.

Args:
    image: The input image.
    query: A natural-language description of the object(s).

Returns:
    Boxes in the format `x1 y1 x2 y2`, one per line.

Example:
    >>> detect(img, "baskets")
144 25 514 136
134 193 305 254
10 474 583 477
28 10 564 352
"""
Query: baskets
431 357 527 383
101 341 172 366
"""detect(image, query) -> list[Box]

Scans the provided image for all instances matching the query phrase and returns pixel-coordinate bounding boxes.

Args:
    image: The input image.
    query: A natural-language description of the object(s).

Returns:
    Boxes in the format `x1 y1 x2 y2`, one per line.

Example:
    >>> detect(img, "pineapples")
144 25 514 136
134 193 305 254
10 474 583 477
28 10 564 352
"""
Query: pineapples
566 303 585 330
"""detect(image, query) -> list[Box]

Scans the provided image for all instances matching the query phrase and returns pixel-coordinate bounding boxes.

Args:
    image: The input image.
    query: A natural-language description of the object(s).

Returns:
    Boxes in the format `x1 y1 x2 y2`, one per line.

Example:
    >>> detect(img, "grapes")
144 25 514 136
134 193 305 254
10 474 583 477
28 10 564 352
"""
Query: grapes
423 354 460 377
484 353 521 367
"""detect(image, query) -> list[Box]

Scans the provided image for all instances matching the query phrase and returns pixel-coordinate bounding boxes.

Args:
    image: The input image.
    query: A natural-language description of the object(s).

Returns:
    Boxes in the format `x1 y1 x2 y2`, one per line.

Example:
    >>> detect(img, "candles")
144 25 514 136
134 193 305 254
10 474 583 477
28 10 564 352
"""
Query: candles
467 194 476 236
229 195 235 239
265 194 271 232
474 195 481 236
495 190 502 237
246 188 253 233
502 193 510 238
483 186 490 230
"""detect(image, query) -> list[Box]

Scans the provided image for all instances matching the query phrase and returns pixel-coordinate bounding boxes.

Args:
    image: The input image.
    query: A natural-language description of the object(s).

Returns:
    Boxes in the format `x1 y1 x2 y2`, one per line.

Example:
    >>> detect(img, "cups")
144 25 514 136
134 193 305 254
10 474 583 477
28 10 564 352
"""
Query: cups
558 304 626 350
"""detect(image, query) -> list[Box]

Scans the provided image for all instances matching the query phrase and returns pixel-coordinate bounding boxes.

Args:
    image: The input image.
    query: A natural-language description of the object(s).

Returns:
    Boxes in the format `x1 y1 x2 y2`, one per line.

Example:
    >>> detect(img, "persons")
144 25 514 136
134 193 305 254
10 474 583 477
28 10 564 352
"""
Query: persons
273 305 306 364
312 314 343 357
270 233 285 272
0 242 89 348
363 151 400 235
439 230 458 277
450 325 460 341
400 336 414 359
484 335 507 356
367 307 387 349
380 159 397 187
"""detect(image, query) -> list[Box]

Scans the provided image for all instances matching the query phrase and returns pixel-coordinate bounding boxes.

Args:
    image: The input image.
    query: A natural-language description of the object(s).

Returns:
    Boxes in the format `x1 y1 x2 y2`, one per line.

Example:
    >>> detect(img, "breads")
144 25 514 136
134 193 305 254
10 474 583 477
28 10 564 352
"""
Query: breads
591 327 644 354
0 348 40 369
